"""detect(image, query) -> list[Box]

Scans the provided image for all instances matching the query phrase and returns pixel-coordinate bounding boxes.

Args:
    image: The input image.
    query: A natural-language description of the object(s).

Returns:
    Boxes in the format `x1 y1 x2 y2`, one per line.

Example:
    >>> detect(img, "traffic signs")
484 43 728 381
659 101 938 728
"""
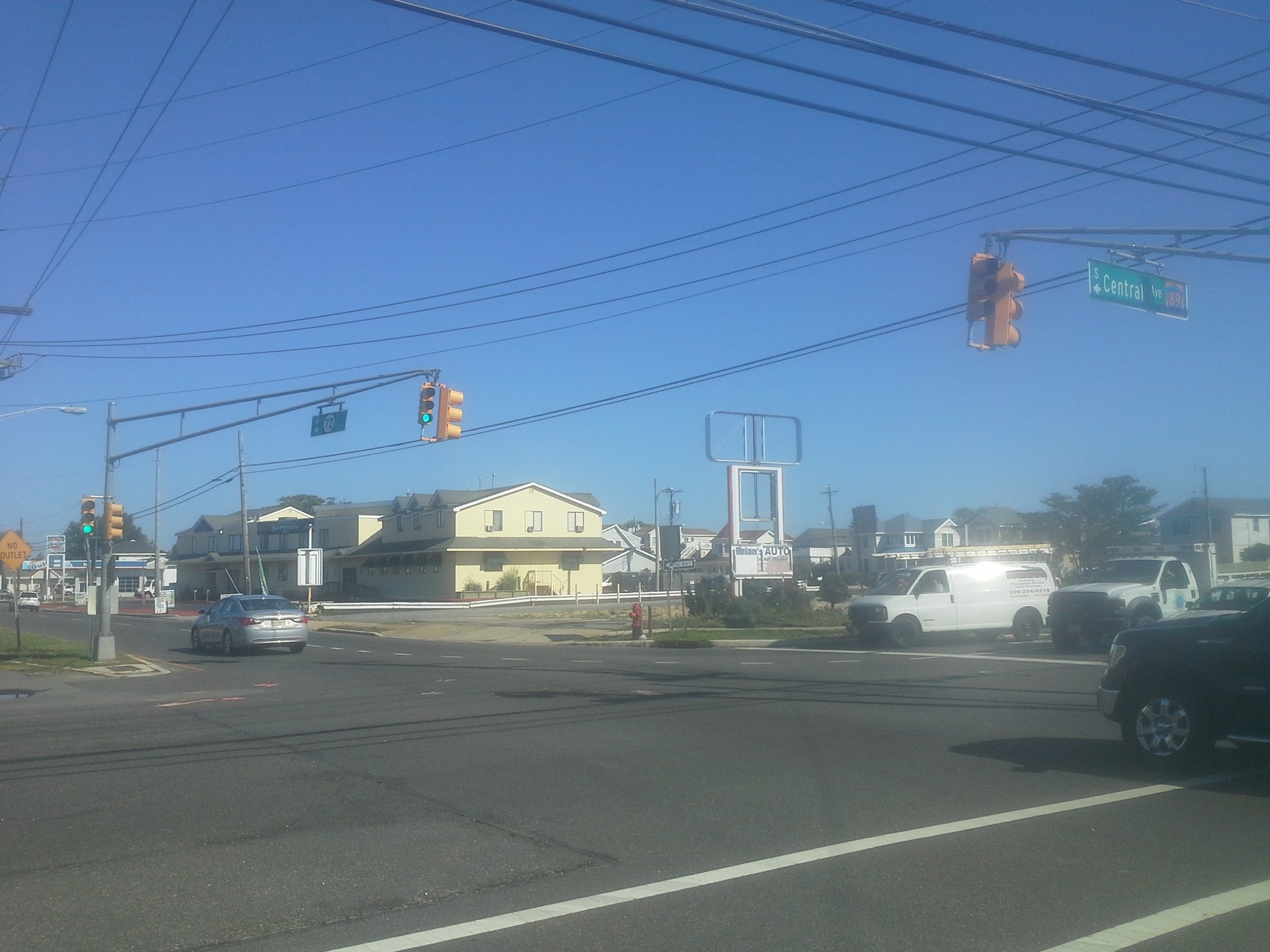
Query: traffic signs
662 559 696 572
1086 258 1190 322
310 409 348 436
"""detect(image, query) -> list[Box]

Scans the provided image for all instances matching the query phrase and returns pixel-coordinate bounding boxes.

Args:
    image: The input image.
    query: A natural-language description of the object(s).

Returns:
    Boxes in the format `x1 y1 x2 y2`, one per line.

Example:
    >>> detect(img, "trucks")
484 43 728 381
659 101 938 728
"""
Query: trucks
1040 542 1270 651
133 567 176 599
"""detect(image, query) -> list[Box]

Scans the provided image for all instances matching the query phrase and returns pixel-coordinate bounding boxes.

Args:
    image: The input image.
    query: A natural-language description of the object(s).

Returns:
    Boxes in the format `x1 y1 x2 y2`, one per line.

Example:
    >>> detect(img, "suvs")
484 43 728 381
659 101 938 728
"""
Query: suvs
1096 591 1270 775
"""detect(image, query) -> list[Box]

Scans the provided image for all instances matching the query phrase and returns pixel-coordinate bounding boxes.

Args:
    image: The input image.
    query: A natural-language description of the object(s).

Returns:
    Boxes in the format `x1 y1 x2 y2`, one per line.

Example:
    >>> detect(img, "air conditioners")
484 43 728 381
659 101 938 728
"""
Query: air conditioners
527 526 535 533
487 526 495 532
575 525 584 533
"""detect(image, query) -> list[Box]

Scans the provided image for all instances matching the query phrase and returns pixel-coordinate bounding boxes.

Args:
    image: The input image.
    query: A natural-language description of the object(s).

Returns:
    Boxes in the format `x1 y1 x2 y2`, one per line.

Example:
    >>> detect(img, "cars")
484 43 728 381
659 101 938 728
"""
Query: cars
8 591 41 613
0 590 12 601
52 583 74 595
189 591 309 655
1158 576 1270 621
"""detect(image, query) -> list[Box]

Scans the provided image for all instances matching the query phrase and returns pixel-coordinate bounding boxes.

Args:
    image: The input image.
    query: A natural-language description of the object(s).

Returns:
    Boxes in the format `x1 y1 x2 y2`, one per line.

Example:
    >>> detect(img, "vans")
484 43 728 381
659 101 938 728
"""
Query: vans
847 561 1058 648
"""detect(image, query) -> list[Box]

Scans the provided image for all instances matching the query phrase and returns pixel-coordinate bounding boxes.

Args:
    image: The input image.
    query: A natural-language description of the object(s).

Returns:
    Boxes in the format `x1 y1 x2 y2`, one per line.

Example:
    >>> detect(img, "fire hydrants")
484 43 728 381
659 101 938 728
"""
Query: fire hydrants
627 603 643 640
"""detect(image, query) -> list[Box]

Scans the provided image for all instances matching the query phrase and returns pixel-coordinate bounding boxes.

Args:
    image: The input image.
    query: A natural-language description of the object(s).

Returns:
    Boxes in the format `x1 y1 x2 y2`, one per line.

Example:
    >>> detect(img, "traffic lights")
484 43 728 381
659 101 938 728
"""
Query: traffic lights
417 384 438 427
105 503 124 540
81 497 94 536
990 263 1026 350
435 385 464 441
968 249 1002 320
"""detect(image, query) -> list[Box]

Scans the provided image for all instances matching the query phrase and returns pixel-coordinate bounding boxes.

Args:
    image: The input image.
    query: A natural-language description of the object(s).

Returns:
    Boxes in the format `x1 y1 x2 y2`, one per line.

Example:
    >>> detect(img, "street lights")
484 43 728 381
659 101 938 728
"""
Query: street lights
1192 487 1214 544
818 520 841 574
653 478 674 592
